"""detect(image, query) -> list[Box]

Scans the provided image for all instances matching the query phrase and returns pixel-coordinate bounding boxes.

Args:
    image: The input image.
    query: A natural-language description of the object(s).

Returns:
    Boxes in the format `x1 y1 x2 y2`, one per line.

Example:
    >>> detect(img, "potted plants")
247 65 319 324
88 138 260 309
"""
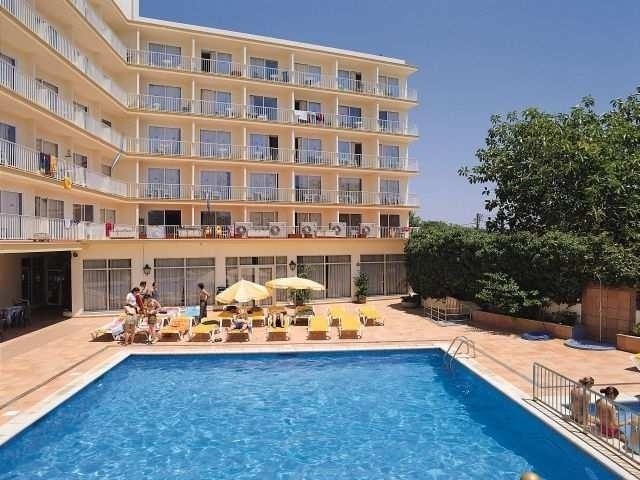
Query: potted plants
288 264 313 306
401 294 420 308
354 272 368 304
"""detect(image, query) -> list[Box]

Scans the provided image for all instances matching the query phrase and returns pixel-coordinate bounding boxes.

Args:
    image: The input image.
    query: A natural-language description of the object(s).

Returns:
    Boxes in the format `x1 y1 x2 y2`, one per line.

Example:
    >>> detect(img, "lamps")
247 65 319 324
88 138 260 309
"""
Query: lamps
143 263 152 276
289 260 296 271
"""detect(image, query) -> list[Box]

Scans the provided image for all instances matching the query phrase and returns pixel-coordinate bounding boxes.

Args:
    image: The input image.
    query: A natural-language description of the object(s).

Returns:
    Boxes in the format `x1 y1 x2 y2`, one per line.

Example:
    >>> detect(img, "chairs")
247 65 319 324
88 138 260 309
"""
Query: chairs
93 303 386 341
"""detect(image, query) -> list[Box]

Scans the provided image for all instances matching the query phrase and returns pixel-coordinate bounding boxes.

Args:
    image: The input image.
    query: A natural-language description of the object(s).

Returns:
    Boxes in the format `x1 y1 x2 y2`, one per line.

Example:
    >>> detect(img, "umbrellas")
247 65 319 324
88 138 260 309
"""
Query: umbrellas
265 277 326 306
214 278 273 314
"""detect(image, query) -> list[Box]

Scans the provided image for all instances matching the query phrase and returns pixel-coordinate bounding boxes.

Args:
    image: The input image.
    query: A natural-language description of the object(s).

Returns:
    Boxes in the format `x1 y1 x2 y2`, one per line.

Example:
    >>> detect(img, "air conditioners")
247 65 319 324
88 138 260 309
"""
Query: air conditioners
235 221 380 238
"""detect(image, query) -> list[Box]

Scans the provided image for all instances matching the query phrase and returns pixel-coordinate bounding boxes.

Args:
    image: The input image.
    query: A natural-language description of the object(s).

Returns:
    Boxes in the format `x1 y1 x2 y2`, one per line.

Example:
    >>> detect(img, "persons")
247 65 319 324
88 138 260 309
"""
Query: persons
520 471 540 480
122 280 162 346
267 310 287 328
196 282 211 326
224 312 248 332
570 375 595 425
627 415 640 455
595 386 631 444
